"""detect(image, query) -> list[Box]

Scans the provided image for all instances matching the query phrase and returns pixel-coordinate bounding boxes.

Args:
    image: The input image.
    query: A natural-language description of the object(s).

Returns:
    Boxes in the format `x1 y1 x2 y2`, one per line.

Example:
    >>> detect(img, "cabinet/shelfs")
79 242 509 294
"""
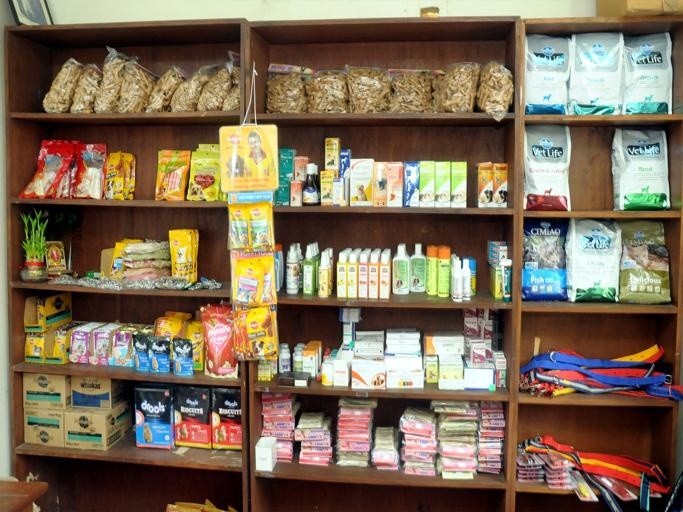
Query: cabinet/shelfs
1 19 681 511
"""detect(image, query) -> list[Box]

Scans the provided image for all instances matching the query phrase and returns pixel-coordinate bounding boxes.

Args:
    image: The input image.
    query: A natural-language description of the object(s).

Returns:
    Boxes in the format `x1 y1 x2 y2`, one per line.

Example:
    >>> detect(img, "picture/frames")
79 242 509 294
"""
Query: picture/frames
9 0 55 28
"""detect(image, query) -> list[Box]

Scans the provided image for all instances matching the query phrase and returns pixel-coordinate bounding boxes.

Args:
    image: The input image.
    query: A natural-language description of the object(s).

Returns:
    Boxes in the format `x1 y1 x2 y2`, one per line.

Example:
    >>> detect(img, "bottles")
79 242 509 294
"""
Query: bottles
301 163 321 207
279 342 304 375
